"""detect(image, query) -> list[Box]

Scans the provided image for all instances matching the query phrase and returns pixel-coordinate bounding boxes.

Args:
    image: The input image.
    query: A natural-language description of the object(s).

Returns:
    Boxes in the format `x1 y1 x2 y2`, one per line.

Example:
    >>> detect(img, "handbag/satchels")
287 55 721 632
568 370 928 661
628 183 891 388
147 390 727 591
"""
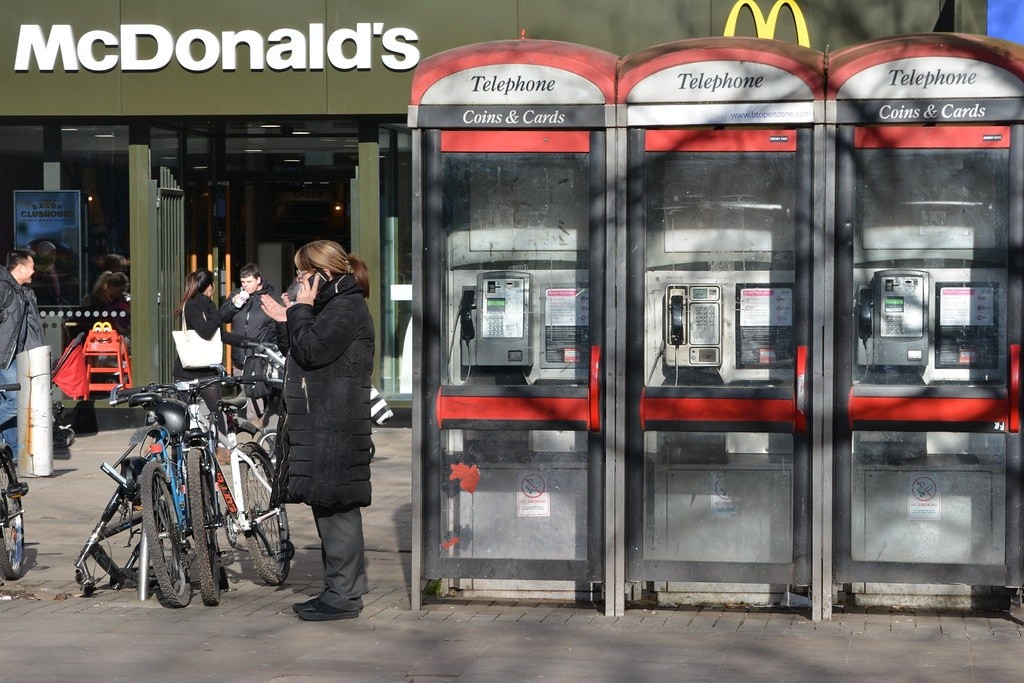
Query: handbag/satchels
171 302 223 369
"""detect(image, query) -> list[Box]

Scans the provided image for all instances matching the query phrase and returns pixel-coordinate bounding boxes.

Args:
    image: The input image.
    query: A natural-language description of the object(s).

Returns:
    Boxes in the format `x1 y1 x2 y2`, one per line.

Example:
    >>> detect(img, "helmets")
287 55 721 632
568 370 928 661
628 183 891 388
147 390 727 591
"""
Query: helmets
146 397 191 436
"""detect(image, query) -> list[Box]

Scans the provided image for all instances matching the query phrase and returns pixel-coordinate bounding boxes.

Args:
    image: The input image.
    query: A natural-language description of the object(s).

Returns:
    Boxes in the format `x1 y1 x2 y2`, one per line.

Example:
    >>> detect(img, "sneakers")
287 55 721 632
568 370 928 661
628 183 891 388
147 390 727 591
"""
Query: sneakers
293 595 321 613
298 602 363 621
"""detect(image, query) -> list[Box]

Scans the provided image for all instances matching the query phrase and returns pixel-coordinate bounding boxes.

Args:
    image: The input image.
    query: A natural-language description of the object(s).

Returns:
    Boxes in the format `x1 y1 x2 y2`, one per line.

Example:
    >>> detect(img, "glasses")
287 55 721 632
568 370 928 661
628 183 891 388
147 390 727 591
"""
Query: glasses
296 270 309 278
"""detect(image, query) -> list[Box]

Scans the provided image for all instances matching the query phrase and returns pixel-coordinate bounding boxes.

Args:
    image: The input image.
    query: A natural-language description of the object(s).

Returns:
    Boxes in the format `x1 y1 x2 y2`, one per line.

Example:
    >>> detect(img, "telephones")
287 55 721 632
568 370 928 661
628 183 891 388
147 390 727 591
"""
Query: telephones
855 268 931 367
456 268 537 367
663 283 723 368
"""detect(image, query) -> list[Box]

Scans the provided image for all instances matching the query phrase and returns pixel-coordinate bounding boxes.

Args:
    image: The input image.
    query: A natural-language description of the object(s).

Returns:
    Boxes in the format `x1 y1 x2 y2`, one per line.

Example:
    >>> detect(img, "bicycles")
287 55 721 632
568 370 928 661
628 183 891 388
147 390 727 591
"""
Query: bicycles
73 340 297 609
0 383 30 579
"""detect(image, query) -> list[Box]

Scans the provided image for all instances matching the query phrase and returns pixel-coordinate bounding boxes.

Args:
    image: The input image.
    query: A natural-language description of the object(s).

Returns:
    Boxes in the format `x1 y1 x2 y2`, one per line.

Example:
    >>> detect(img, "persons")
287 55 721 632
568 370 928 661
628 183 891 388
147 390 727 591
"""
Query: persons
32 243 131 338
260 240 377 621
0 248 46 468
173 262 285 436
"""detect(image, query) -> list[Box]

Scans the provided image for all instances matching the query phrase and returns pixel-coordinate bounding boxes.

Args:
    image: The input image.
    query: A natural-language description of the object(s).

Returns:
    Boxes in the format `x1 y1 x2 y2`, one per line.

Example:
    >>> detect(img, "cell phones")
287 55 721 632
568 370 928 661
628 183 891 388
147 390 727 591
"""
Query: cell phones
308 269 330 291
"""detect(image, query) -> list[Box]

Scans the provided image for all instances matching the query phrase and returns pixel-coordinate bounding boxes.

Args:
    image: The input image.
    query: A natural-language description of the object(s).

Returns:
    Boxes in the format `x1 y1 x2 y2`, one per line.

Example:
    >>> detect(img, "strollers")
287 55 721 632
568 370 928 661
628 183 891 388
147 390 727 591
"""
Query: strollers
46 332 89 447
